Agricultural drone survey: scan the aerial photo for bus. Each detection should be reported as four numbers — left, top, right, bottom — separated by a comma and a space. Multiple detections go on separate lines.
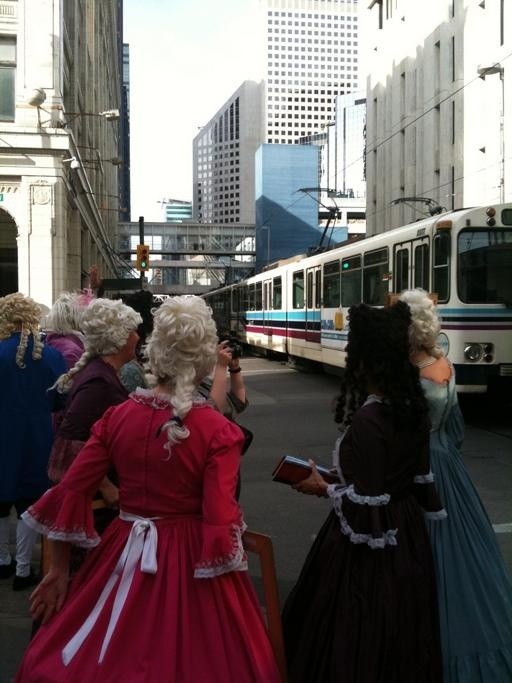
198, 200, 511, 396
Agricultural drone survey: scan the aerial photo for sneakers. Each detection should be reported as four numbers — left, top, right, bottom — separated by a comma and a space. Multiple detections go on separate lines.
0, 557, 16, 579
13, 571, 39, 591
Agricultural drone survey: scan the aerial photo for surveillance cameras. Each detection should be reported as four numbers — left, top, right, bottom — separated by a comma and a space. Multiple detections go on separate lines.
70, 160, 80, 171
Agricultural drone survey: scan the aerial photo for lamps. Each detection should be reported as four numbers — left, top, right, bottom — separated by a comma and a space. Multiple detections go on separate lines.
80, 153, 124, 166
25, 86, 47, 126
63, 107, 121, 121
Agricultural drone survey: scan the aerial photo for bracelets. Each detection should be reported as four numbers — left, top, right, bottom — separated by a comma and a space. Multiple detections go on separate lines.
228, 366, 242, 374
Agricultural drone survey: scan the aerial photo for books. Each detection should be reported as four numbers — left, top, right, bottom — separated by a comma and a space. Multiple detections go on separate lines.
271, 454, 343, 491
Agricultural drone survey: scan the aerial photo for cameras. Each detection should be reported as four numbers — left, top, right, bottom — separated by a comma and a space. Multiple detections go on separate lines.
226, 343, 243, 357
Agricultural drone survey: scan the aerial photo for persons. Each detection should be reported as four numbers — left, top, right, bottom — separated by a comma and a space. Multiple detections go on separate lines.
17, 290, 291, 683
41, 291, 88, 384
386, 283, 511, 683
42, 297, 144, 574
116, 317, 148, 397
197, 334, 252, 503
280, 298, 449, 681
2, 289, 49, 593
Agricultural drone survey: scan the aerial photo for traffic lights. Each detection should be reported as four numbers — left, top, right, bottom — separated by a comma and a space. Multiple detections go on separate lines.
137, 244, 148, 269
